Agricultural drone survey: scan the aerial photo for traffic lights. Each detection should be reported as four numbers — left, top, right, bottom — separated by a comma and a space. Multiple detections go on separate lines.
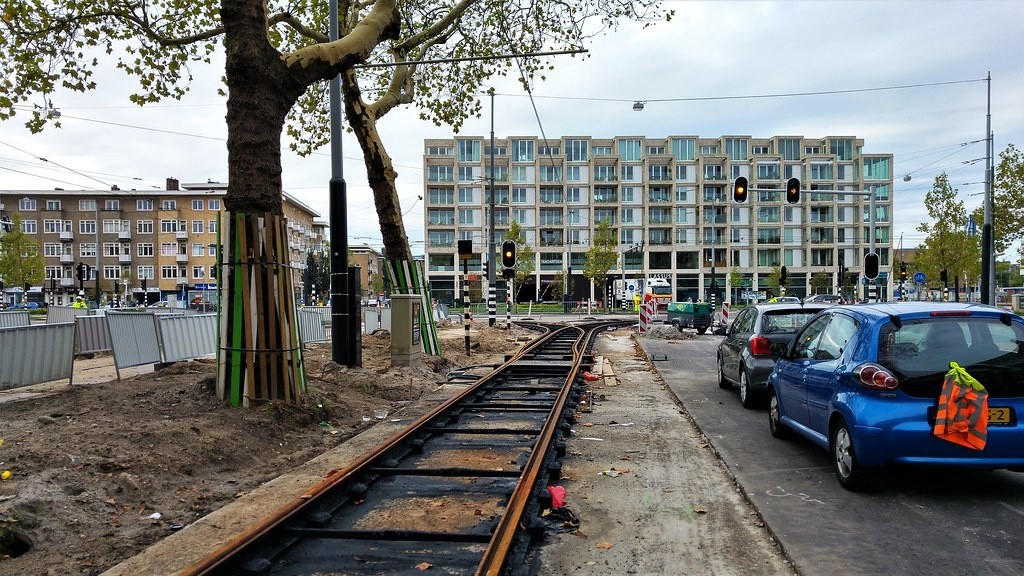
900, 266, 906, 280
458, 240, 472, 254
141, 280, 146, 290
502, 240, 516, 268
941, 270, 947, 281
734, 176, 748, 204
625, 281, 628, 290
864, 252, 881, 281
115, 283, 119, 292
787, 177, 800, 205
82, 264, 91, 281
483, 260, 488, 280
1, 215, 11, 232
640, 239, 645, 253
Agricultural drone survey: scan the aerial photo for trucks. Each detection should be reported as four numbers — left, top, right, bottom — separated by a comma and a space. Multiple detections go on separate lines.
612, 276, 673, 312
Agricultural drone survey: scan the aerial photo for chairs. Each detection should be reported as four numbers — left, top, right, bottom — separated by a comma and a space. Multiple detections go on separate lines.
925, 322, 967, 348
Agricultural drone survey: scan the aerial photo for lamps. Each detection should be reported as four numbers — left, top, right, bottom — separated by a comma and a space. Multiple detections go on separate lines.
632, 101, 644, 111
904, 174, 912, 182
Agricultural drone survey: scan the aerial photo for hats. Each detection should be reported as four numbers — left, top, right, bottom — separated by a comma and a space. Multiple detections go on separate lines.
76, 296, 82, 299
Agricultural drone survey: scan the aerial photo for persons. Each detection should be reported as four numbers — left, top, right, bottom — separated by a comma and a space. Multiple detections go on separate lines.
73, 296, 87, 308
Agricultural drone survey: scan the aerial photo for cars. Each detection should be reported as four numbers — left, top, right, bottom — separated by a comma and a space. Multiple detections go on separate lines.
64, 297, 222, 313
766, 301, 1024, 493
712, 301, 838, 409
361, 292, 391, 308
802, 293, 848, 305
761, 297, 801, 305
2, 302, 48, 310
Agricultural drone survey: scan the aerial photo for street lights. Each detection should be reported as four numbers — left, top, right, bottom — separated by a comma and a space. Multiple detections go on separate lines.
488, 86, 495, 325
